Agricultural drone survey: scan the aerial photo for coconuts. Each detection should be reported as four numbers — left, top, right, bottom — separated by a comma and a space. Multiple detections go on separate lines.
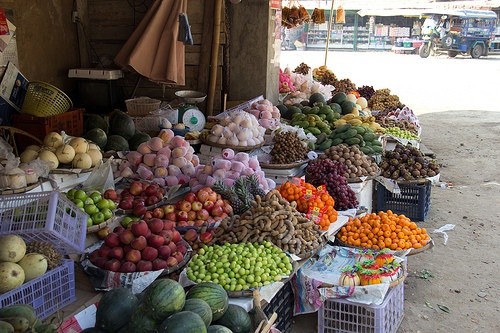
276, 92, 354, 120
81, 110, 164, 152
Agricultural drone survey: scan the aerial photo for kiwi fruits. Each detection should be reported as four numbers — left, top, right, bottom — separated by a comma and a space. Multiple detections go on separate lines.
314, 144, 376, 179
374, 115, 419, 134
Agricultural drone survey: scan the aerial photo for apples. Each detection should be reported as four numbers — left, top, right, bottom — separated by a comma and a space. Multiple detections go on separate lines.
206, 99, 281, 146
68, 180, 233, 272
113, 129, 275, 194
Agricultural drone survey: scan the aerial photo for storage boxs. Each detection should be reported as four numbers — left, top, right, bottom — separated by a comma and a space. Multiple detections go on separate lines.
263, 279, 295, 333
199, 143, 225, 158
186, 139, 202, 154
0, 258, 76, 321
412, 29, 421, 36
394, 40, 404, 47
413, 41, 423, 47
317, 278, 406, 333
74, 78, 120, 114
318, 21, 329, 31
10, 108, 87, 148
371, 180, 432, 223
260, 161, 308, 185
334, 23, 343, 30
67, 68, 122, 81
0, 190, 90, 257
296, 43, 307, 51
403, 41, 413, 47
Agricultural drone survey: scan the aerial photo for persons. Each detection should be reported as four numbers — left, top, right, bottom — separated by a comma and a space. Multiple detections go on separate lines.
437, 19, 450, 39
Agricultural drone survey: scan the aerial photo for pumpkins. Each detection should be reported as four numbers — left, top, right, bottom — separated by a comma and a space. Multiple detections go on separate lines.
339, 248, 402, 286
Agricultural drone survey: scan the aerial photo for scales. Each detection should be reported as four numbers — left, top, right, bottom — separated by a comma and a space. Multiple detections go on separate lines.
174, 90, 207, 132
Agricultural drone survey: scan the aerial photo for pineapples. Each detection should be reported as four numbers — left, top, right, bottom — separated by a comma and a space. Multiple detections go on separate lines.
213, 175, 265, 213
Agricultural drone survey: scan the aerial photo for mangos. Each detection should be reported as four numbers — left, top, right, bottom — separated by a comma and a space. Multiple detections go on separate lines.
290, 112, 385, 154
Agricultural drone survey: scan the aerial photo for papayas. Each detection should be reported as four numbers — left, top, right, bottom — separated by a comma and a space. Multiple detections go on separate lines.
0, 305, 64, 333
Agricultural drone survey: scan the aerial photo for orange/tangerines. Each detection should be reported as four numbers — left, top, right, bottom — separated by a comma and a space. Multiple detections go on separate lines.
276, 179, 338, 230
337, 210, 429, 250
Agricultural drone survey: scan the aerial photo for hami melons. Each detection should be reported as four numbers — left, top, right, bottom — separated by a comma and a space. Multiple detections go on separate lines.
0, 235, 48, 294
5, 132, 103, 188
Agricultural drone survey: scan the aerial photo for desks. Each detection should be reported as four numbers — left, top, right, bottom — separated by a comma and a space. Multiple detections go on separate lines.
228, 214, 350, 312
347, 142, 397, 214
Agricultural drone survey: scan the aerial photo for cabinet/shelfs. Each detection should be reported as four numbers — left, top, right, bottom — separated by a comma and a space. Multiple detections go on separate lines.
342, 29, 354, 49
306, 29, 342, 49
357, 28, 369, 50
369, 35, 412, 52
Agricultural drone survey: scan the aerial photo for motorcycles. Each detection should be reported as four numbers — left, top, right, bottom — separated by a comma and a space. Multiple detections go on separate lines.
418, 9, 498, 59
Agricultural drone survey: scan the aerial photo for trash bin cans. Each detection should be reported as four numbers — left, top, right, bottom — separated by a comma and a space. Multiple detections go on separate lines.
22, 81, 73, 117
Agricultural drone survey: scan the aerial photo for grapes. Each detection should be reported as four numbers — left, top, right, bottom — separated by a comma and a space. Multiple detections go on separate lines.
304, 160, 359, 211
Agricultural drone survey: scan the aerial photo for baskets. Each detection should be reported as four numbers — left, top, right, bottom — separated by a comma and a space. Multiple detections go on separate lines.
0, 191, 89, 254
0, 259, 77, 321
20, 81, 74, 119
250, 279, 294, 333
125, 98, 161, 116
378, 181, 431, 222
317, 281, 406, 333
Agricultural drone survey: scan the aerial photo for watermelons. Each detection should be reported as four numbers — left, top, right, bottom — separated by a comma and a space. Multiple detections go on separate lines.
80, 279, 252, 333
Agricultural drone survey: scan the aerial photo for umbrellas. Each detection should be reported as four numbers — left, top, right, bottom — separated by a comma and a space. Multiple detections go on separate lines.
113, 0, 187, 99
